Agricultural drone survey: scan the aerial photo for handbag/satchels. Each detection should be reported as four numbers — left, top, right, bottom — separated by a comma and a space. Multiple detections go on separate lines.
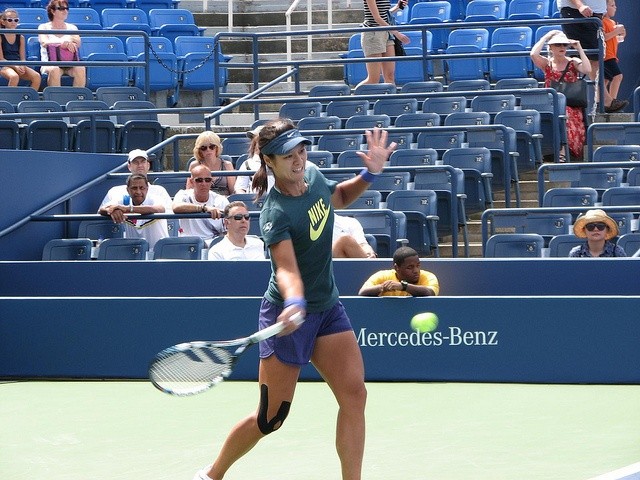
387, 10, 407, 56
550, 60, 587, 108
47, 44, 80, 61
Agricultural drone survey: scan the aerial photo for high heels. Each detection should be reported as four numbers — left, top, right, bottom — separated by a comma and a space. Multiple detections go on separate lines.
559, 154, 566, 162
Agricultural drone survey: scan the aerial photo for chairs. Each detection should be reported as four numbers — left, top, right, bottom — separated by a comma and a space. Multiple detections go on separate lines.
373, 98, 416, 115
318, 135, 364, 151
396, 113, 440, 128
133, 0, 177, 17
410, 1, 452, 24
325, 174, 356, 183
97, 238, 148, 260
467, 127, 522, 209
617, 234, 640, 257
9, 8, 46, 38
306, 151, 332, 167
96, 86, 145, 107
534, 26, 569, 80
252, 119, 274, 128
227, 195, 266, 236
400, 82, 442, 93
43, 87, 92, 106
493, 79, 538, 90
308, 85, 349, 97
64, 8, 102, 37
326, 100, 368, 118
362, 234, 377, 257
73, 119, 119, 155
348, 191, 380, 210
297, 117, 341, 130
0, 1, 30, 12
405, 141, 423, 143
391, 3, 409, 25
0, 86, 40, 104
447, 80, 491, 91
444, 111, 491, 126
345, 115, 390, 128
442, 148, 494, 209
388, 131, 414, 151
394, 30, 432, 85
27, 120, 68, 152
490, 27, 533, 78
166, 219, 179, 237
602, 187, 640, 205
549, 234, 588, 258
515, 213, 573, 237
388, 149, 437, 169
386, 190, 438, 259
445, 28, 488, 79
423, 97, 466, 114
101, 9, 151, 55
576, 212, 634, 235
417, 132, 463, 150
123, 121, 162, 172
465, 0, 507, 21
65, 100, 109, 125
152, 235, 205, 260
0, 120, 19, 150
220, 139, 251, 156
187, 155, 232, 170
552, 1, 560, 17
26, 37, 75, 92
208, 234, 260, 248
303, 135, 315, 145
592, 144, 640, 163
484, 233, 543, 259
508, 0, 550, 20
235, 154, 251, 170
114, 101, 158, 126
493, 109, 543, 166
340, 32, 368, 84
354, 211, 406, 250
471, 95, 513, 112
78, 219, 123, 241
40, 1, 79, 9
177, 36, 228, 90
0, 100, 15, 122
77, 37, 129, 87
18, 100, 62, 124
355, 82, 397, 96
368, 172, 411, 190
124, 36, 177, 91
339, 151, 366, 169
569, 168, 623, 186
626, 166, 640, 186
321, 151, 323, 154
279, 104, 321, 120
42, 237, 92, 261
152, 176, 189, 202
520, 92, 570, 166
542, 186, 598, 207
149, 8, 199, 50
90, 0, 127, 14
414, 169, 470, 258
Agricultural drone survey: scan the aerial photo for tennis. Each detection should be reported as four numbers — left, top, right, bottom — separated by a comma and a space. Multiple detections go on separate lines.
410, 311, 439, 335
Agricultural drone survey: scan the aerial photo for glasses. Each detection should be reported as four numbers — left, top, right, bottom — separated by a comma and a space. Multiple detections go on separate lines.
227, 213, 249, 220
3, 18, 19, 22
585, 223, 608, 231
551, 44, 567, 47
199, 145, 216, 151
194, 177, 212, 182
55, 6, 69, 11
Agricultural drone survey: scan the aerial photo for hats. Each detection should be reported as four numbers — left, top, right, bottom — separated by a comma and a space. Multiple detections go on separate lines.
128, 149, 148, 164
573, 210, 619, 240
260, 127, 312, 155
548, 31, 571, 44
247, 125, 265, 138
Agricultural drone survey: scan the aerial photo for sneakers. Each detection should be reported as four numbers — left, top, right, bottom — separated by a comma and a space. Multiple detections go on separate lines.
604, 98, 628, 112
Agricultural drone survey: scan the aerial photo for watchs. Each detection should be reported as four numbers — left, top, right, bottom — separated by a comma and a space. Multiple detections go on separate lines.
402, 279, 408, 290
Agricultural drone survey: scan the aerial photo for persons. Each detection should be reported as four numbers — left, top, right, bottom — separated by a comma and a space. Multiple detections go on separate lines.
208, 202, 264, 260
190, 131, 236, 194
328, 212, 373, 254
99, 150, 172, 213
359, 248, 439, 298
571, 209, 627, 257
531, 31, 593, 157
0, 12, 41, 91
599, 0, 624, 105
39, 1, 85, 88
238, 126, 275, 194
354, 0, 409, 87
105, 176, 173, 245
557, 2, 625, 111
193, 121, 396, 480
169, 166, 233, 239
